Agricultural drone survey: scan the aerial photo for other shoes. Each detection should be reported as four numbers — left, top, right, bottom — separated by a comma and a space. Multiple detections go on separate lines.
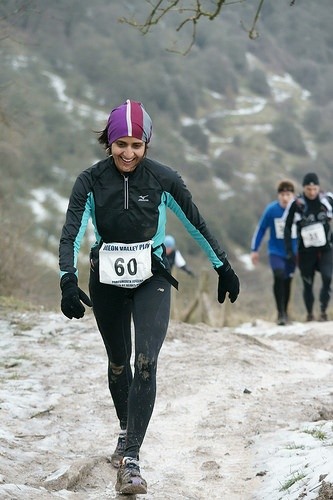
277, 313, 285, 325
321, 307, 328, 321
307, 313, 312, 321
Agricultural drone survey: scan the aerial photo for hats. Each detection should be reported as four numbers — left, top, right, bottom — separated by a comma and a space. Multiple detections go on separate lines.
303, 173, 320, 186
164, 234, 176, 248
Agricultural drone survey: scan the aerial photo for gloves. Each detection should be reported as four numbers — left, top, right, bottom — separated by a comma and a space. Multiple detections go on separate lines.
287, 250, 296, 260
60, 274, 95, 319
214, 261, 240, 304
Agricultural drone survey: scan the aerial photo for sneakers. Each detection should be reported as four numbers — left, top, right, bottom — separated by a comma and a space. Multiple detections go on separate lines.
111, 437, 126, 468
115, 463, 147, 494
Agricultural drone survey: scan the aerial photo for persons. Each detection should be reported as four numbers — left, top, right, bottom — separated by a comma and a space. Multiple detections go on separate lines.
58, 99, 240, 494
281, 172, 333, 322
165, 235, 192, 275
250, 180, 299, 324
